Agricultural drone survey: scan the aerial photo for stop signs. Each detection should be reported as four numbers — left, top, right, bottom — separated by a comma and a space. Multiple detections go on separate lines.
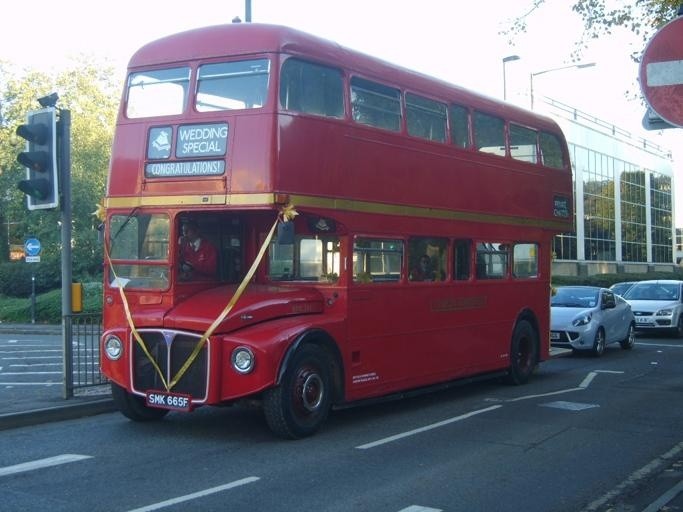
640, 15, 682, 126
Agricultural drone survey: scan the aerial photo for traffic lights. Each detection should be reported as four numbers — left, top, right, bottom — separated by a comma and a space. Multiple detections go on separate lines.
16, 106, 61, 212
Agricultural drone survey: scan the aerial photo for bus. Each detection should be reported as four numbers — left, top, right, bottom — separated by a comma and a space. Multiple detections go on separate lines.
98, 17, 574, 440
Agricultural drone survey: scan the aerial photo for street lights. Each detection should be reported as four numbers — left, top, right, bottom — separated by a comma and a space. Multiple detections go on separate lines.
503, 55, 595, 113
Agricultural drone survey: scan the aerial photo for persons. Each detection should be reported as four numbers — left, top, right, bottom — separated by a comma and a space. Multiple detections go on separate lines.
409, 254, 444, 280
176, 221, 217, 280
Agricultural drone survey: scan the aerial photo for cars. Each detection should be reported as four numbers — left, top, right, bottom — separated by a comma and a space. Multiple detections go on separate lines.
551, 279, 683, 357
